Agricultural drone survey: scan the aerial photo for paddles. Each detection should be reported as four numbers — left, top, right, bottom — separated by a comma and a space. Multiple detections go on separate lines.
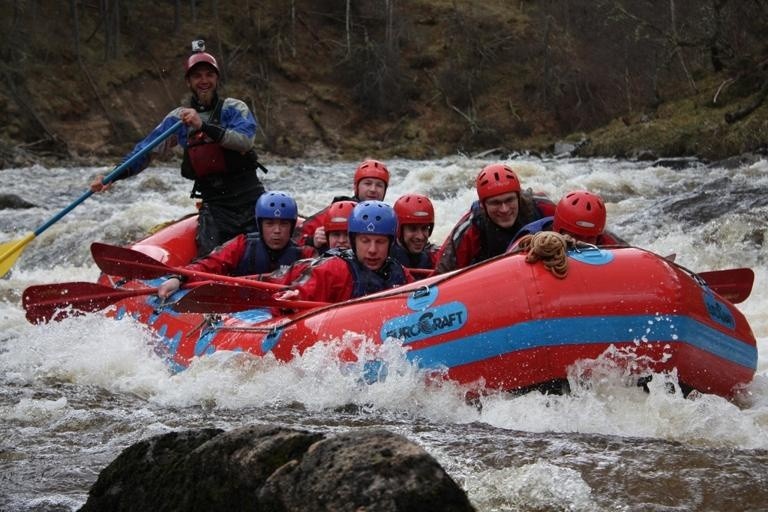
695, 265, 754, 304
22, 272, 211, 328
0, 117, 189, 269
88, 242, 285, 291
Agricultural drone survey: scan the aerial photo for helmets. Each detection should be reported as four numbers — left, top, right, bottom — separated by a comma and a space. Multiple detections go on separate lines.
347, 200, 399, 256
476, 165, 521, 202
185, 52, 220, 74
552, 192, 606, 237
354, 160, 389, 196
255, 191, 297, 239
393, 195, 434, 247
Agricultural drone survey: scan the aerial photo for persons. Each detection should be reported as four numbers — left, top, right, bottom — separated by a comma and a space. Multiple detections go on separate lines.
428, 162, 557, 277
270, 199, 417, 318
388, 194, 443, 280
157, 189, 320, 301
290, 159, 391, 257
89, 50, 266, 259
505, 190, 630, 253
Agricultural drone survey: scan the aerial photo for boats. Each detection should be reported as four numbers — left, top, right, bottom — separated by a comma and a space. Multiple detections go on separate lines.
97, 212, 758, 414
